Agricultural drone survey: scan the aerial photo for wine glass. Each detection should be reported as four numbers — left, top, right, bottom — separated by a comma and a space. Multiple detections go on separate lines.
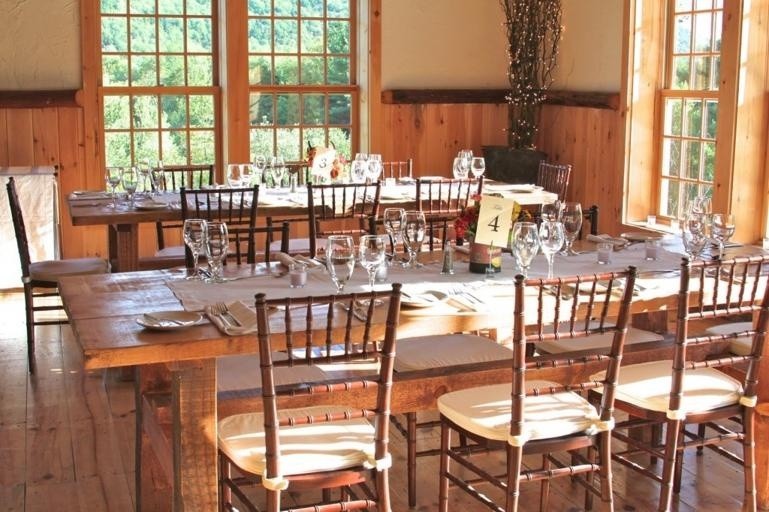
510, 197, 583, 290
227, 156, 285, 203
452, 149, 485, 186
104, 158, 164, 209
682, 213, 736, 275
358, 235, 385, 307
382, 208, 426, 270
351, 153, 383, 193
326, 235, 356, 295
183, 219, 229, 283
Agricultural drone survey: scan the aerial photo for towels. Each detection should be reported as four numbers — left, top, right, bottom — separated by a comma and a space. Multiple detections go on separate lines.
205, 301, 258, 335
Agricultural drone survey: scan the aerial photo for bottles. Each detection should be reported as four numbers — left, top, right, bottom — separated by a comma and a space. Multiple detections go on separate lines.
288, 175, 295, 192
441, 240, 454, 275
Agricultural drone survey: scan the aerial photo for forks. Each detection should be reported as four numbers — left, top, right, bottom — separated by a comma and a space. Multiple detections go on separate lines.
210, 300, 242, 327
448, 287, 485, 305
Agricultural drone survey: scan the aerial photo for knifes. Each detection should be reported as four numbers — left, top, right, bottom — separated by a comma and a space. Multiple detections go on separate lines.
337, 301, 367, 323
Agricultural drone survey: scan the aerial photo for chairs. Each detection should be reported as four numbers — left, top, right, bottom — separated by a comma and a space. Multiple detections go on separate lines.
184, 220, 292, 264
6, 176, 110, 373
588, 256, 769, 512
515, 205, 659, 480
368, 216, 514, 504
436, 266, 635, 512
218, 282, 402, 512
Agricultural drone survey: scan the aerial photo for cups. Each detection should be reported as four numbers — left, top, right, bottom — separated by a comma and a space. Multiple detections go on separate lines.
596, 242, 613, 264
647, 215, 656, 225
645, 240, 660, 261
289, 263, 308, 288
671, 220, 679, 230
386, 178, 395, 190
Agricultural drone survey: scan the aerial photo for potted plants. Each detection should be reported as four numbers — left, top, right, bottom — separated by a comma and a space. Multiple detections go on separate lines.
482, 0, 565, 185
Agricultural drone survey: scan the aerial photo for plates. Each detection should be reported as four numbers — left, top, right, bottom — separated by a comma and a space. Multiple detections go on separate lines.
400, 290, 450, 308
135, 311, 203, 331
620, 232, 663, 241
